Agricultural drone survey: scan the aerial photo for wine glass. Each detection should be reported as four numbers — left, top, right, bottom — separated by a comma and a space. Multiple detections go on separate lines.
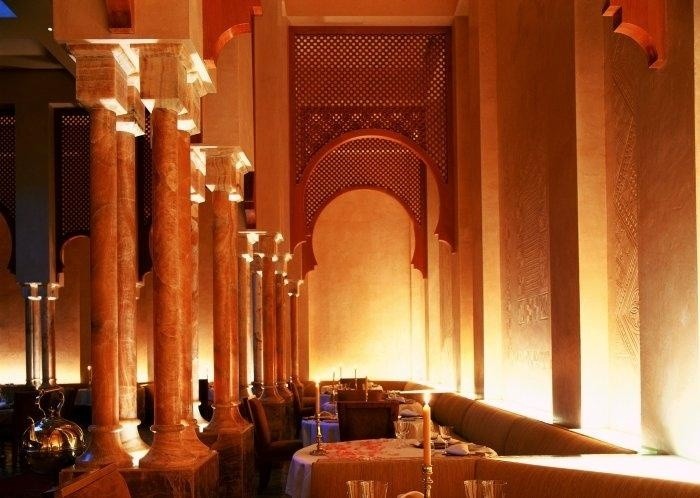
439, 425, 454, 456
393, 420, 411, 449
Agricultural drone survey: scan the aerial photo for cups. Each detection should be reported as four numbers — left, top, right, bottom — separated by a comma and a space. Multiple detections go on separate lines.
345, 480, 389, 498
463, 478, 509, 498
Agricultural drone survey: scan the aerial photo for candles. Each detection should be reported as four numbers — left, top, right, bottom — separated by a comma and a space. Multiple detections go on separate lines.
419, 390, 432, 467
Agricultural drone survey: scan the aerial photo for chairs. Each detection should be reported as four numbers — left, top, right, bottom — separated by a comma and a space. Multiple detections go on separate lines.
241, 375, 400, 498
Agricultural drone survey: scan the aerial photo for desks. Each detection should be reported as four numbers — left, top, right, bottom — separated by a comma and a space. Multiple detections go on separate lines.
290, 439, 498, 498
